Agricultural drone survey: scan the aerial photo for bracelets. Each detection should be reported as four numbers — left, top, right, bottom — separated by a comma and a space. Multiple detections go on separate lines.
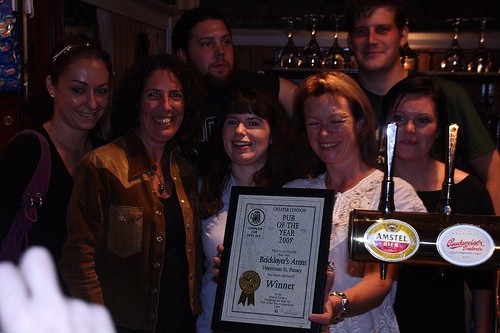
330, 291, 350, 325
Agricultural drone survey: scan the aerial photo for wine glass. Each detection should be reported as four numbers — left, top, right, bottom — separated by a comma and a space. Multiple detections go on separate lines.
445, 18, 468, 72
323, 14, 348, 69
301, 15, 325, 68
400, 18, 417, 70
279, 17, 302, 68
471, 17, 494, 73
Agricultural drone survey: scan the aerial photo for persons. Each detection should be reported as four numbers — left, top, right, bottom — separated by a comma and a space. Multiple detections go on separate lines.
345, 0, 500, 216
376, 77, 496, 333
195, 87, 297, 333
171, 8, 299, 145
212, 71, 429, 333
60, 54, 202, 332
0, 34, 113, 299
